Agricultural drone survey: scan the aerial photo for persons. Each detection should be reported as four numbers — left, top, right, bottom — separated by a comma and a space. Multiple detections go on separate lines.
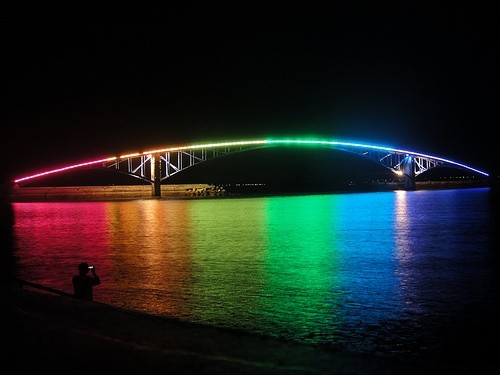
72, 262, 102, 301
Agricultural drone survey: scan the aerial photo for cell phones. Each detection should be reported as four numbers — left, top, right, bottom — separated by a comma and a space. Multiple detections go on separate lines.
88, 266, 94, 270
